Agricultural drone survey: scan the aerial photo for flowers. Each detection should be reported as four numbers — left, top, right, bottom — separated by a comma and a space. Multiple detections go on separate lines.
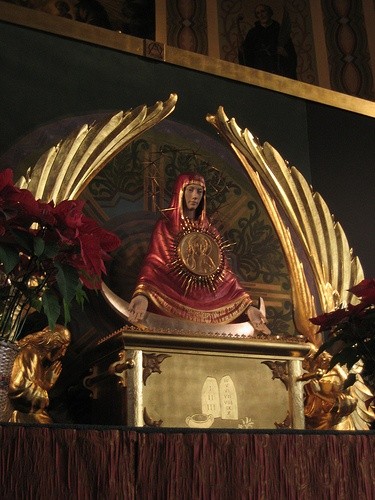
308, 277, 375, 431
0, 168, 124, 344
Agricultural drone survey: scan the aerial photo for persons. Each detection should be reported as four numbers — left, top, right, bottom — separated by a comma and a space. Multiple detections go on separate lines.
126, 173, 272, 336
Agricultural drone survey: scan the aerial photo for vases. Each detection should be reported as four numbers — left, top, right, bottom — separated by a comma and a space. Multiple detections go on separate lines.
0, 323, 71, 425
